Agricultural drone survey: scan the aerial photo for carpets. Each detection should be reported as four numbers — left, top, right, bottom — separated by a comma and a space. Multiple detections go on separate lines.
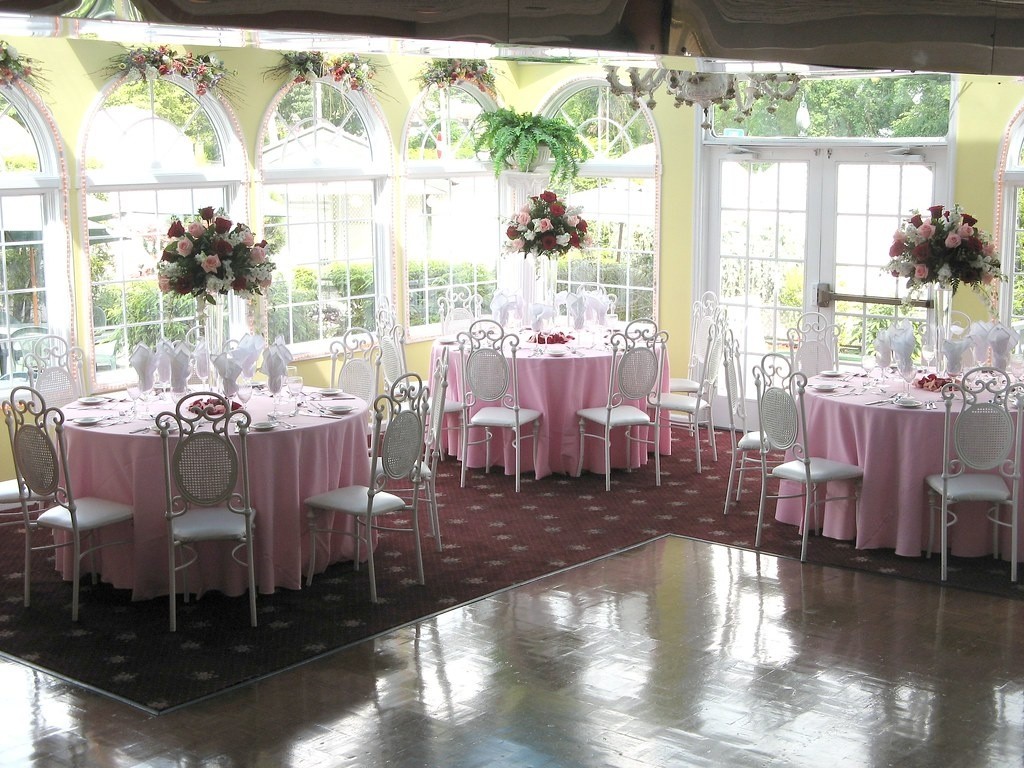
0, 427, 1021, 718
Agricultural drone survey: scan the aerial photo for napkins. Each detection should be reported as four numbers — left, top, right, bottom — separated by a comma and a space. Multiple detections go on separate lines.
129, 287, 1022, 395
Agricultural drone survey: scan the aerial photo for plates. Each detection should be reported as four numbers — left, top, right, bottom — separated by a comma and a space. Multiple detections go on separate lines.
327, 404, 352, 415
895, 398, 922, 409
148, 423, 177, 433
249, 422, 278, 431
72, 416, 101, 426
548, 351, 566, 356
78, 396, 104, 405
319, 388, 344, 396
812, 384, 838, 392
440, 337, 457, 344
821, 370, 842, 376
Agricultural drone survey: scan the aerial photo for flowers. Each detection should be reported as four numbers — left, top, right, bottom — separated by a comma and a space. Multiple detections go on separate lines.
86, 42, 247, 108
157, 205, 277, 305
885, 201, 1010, 305
260, 50, 399, 103
410, 57, 506, 104
496, 191, 593, 257
0, 40, 53, 94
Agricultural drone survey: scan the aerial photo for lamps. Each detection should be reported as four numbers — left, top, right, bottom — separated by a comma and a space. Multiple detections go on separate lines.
604, 65, 805, 129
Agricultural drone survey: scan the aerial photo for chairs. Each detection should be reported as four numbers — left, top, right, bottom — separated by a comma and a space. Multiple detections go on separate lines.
25, 336, 86, 434
329, 283, 1024, 633
2, 386, 134, 622
305, 374, 428, 588
155, 391, 259, 631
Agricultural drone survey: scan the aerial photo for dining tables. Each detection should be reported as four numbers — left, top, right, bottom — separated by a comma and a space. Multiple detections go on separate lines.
775, 366, 1024, 566
426, 319, 672, 480
50, 380, 370, 604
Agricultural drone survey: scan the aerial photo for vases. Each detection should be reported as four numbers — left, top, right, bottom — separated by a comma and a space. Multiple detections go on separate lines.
526, 247, 558, 324
200, 287, 227, 392
925, 282, 954, 374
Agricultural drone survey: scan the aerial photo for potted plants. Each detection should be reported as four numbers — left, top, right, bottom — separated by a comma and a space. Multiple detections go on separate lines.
470, 106, 590, 187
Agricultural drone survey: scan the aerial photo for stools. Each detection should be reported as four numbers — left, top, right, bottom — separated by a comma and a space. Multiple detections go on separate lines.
0, 477, 55, 533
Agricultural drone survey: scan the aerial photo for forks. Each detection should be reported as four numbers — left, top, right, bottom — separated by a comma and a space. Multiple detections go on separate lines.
812, 369, 938, 410
65, 391, 355, 434
438, 324, 586, 357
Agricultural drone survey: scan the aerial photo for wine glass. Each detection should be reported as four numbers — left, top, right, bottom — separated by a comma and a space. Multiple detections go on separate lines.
125, 367, 304, 422
490, 308, 618, 351
862, 346, 1023, 403
